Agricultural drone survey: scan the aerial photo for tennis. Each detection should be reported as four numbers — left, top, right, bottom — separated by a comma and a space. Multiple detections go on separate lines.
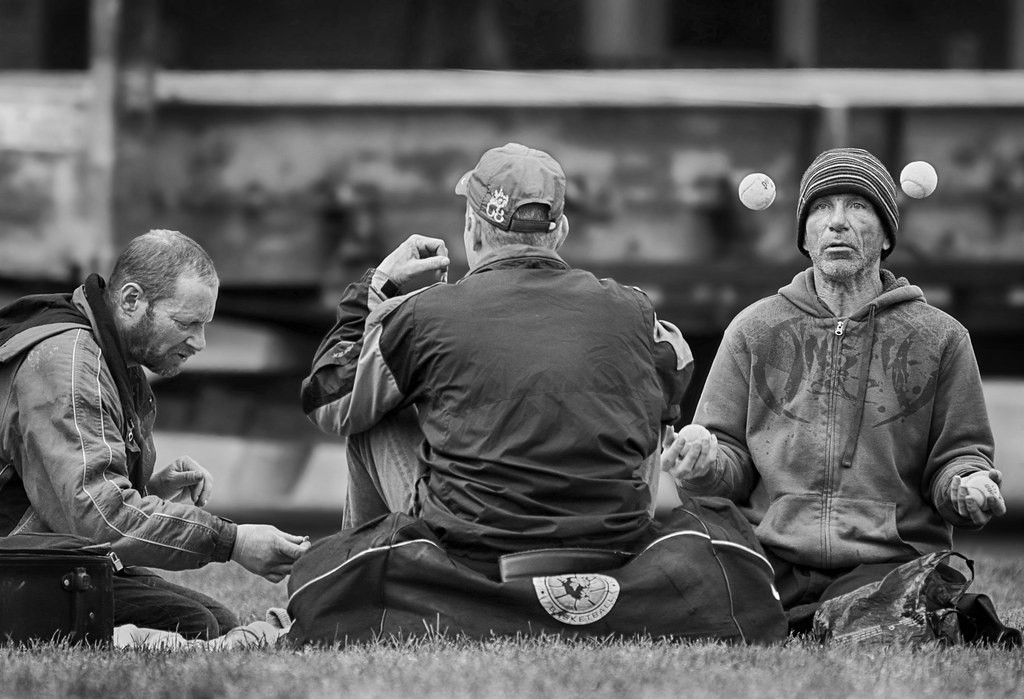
738, 171, 776, 211
963, 476, 1000, 510
899, 160, 939, 199
677, 423, 712, 459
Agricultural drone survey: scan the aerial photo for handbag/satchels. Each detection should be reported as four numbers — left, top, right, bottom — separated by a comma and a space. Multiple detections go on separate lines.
0, 533, 122, 649
288, 496, 789, 648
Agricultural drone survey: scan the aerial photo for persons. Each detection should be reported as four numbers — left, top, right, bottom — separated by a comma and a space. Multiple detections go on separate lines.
0, 228, 313, 650
301, 143, 697, 579
659, 147, 1007, 637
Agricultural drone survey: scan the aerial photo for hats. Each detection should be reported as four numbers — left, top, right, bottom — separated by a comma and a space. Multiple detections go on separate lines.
795, 146, 900, 261
455, 143, 567, 233
